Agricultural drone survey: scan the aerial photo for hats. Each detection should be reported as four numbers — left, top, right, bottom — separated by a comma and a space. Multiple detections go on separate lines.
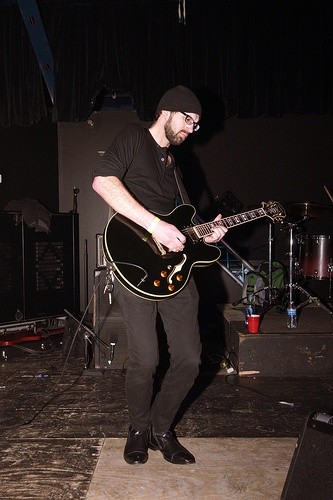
157, 86, 202, 116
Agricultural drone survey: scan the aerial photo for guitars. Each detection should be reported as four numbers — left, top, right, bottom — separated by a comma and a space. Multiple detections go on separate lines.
103, 200, 286, 301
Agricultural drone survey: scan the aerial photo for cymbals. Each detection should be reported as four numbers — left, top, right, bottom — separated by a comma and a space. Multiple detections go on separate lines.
287, 203, 333, 218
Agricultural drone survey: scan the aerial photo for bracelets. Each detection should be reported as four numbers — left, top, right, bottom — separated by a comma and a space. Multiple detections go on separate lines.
147, 217, 161, 233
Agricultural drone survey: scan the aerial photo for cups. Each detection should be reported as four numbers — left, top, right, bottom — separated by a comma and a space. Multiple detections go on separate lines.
247, 314, 260, 333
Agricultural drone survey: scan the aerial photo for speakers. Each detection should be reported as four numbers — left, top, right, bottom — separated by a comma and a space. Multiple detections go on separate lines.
94, 269, 162, 371
280, 411, 333, 500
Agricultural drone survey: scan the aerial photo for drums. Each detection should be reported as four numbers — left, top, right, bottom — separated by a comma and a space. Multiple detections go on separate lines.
292, 234, 333, 280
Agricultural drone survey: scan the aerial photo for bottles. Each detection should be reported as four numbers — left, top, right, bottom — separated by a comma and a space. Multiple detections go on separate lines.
244, 295, 256, 325
287, 301, 297, 328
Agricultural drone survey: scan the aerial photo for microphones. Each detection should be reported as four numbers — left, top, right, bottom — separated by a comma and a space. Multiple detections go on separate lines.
110, 335, 117, 359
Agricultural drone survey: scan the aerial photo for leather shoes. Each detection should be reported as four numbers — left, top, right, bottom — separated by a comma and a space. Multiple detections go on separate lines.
148, 432, 196, 464
124, 424, 148, 465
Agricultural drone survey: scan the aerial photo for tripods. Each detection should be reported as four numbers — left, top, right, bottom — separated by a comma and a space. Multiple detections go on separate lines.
235, 223, 333, 316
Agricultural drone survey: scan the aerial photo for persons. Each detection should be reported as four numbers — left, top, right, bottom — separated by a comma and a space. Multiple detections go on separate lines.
92, 86, 227, 464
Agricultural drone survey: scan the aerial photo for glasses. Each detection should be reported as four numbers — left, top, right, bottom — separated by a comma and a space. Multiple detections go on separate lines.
180, 111, 200, 132
